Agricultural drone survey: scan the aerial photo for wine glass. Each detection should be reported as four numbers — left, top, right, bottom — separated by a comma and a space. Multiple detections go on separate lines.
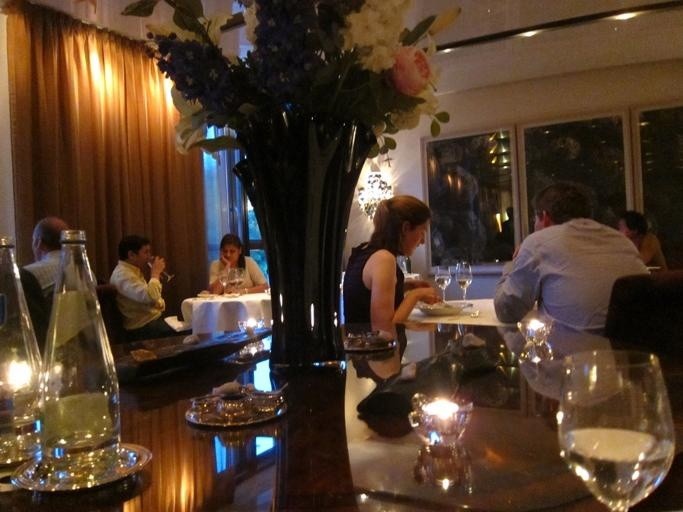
219, 268, 245, 298
434, 265, 452, 301
455, 263, 473, 301
147, 256, 175, 283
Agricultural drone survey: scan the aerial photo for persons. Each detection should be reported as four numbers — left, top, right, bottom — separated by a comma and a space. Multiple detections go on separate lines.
0, 217, 96, 355
615, 211, 668, 275
343, 323, 440, 398
484, 178, 652, 337
497, 325, 628, 432
341, 192, 443, 326
107, 233, 193, 338
207, 232, 269, 296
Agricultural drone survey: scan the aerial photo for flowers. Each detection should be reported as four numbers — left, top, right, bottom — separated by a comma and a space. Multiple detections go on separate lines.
119, 1, 465, 168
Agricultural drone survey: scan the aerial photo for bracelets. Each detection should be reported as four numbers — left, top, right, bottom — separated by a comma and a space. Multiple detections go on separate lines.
243, 287, 248, 293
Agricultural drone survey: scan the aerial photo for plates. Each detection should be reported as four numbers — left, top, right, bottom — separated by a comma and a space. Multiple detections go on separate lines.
185, 396, 288, 427
197, 294, 217, 299
343, 336, 396, 350
10, 442, 153, 492
416, 302, 473, 316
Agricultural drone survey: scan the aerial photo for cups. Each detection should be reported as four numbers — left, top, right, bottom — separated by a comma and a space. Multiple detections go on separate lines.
556, 348, 676, 512
407, 391, 474, 449
517, 319, 556, 347
192, 390, 284, 422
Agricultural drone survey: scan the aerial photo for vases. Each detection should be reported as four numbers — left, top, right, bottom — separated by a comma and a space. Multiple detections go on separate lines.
231, 109, 378, 376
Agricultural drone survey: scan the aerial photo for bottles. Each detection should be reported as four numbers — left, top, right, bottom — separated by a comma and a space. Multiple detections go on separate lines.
0, 230, 121, 481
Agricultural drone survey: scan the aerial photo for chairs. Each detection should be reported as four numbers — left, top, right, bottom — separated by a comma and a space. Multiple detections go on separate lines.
94, 286, 168, 338
606, 274, 683, 361
